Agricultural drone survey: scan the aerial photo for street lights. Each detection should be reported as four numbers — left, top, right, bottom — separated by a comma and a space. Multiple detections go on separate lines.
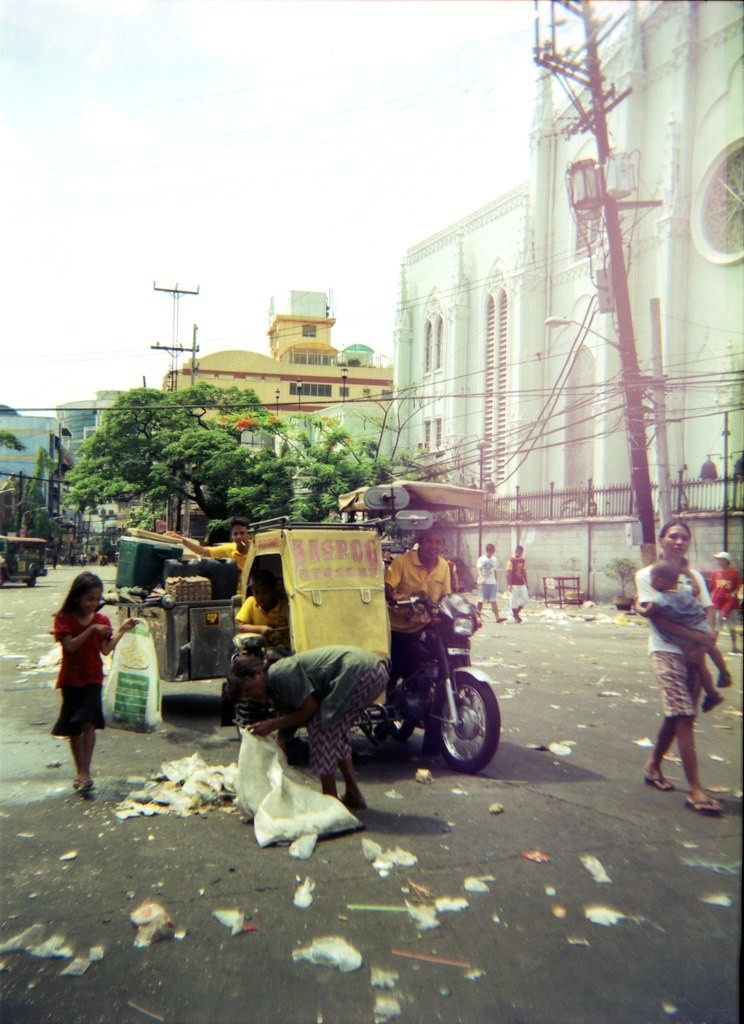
545, 316, 658, 566
342, 369, 348, 404
296, 378, 302, 411
275, 388, 280, 416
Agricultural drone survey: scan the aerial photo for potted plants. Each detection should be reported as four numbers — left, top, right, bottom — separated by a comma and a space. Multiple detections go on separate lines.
605, 559, 636, 611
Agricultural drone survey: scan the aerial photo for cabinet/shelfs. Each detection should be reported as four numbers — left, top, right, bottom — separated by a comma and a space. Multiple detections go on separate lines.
542, 576, 581, 610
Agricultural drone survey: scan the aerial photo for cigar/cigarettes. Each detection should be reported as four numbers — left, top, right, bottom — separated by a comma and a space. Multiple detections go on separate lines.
240, 540, 246, 547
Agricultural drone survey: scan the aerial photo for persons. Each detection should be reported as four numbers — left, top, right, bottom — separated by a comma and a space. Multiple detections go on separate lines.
706, 551, 742, 653
234, 567, 290, 633
51, 573, 140, 794
634, 520, 723, 815
6, 541, 40, 575
382, 527, 460, 692
505, 546, 528, 624
225, 645, 390, 810
42, 543, 119, 569
632, 561, 732, 712
476, 544, 507, 623
163, 516, 254, 596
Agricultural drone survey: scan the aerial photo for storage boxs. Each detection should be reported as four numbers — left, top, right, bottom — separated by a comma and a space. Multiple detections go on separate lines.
115, 541, 184, 588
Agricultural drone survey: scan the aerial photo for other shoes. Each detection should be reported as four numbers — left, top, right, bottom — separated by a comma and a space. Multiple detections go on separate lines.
396, 683, 420, 708
717, 671, 732, 688
497, 618, 507, 623
73, 779, 94, 798
729, 648, 742, 656
514, 614, 521, 622
702, 691, 724, 713
338, 793, 367, 809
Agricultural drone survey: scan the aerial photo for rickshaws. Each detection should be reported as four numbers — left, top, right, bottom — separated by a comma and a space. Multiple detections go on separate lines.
229, 480, 502, 773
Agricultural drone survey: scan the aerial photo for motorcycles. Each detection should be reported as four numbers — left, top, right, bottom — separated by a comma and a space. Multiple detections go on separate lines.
0, 536, 48, 587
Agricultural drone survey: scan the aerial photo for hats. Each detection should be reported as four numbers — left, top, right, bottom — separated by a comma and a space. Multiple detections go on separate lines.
714, 552, 731, 560
418, 523, 446, 545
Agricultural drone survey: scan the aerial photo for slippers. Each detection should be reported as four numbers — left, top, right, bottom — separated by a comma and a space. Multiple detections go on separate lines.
643, 773, 674, 791
685, 797, 723, 816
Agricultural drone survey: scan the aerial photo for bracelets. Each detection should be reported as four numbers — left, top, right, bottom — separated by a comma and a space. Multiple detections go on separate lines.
269, 719, 275, 731
